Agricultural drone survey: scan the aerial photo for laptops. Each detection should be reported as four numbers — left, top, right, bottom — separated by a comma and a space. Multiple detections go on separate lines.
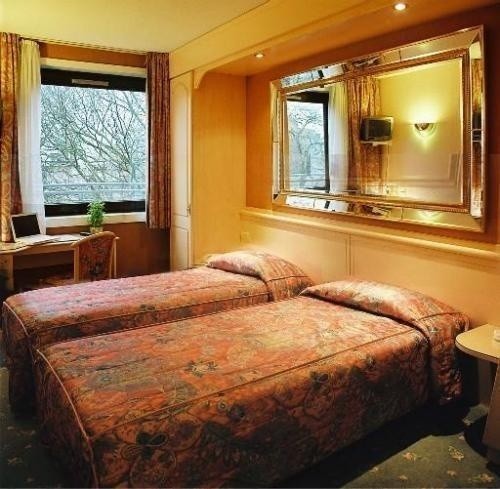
10, 213, 58, 247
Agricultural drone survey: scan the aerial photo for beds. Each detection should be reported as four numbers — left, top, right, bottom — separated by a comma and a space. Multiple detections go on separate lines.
33, 277, 471, 487
3, 252, 314, 401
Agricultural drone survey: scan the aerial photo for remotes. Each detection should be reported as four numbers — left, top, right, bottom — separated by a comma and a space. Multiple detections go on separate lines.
80, 231, 91, 236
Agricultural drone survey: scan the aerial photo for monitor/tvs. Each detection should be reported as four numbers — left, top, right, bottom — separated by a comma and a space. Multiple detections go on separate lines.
361, 116, 393, 142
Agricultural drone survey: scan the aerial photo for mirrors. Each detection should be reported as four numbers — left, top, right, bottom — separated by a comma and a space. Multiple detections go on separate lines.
268, 24, 487, 235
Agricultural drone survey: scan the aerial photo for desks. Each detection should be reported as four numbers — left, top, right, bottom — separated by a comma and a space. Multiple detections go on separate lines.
0, 234, 120, 290
454, 323, 499, 466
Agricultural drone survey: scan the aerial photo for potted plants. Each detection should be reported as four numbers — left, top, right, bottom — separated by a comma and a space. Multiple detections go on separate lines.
86, 198, 106, 233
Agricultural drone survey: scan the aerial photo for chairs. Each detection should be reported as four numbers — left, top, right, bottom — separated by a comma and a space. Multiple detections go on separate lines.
71, 231, 120, 284
